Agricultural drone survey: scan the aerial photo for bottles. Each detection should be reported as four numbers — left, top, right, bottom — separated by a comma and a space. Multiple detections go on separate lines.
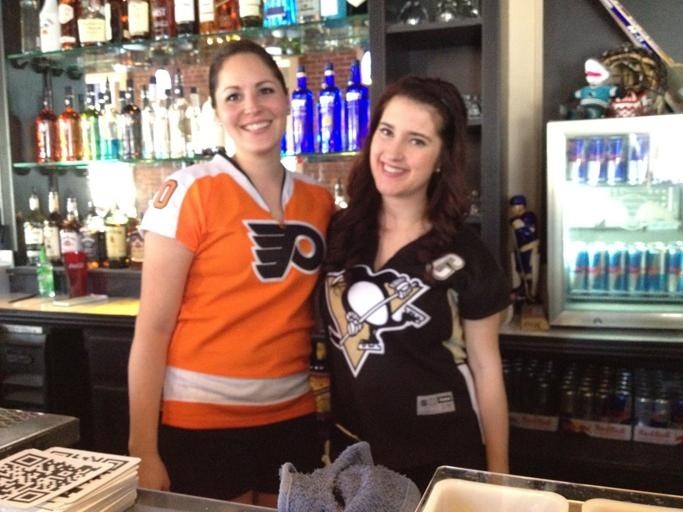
80, 84, 100, 160
128, 0, 347, 38
78, 0, 107, 47
18, 0, 41, 55
119, 76, 225, 160
60, 0, 82, 49
35, 246, 56, 298
23, 187, 146, 269
38, 0, 60, 53
96, 92, 119, 160
289, 61, 369, 152
34, 87, 56, 162
57, 86, 81, 161
105, 0, 130, 43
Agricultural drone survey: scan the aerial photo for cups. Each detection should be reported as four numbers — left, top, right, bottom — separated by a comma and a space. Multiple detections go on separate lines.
63, 252, 88, 298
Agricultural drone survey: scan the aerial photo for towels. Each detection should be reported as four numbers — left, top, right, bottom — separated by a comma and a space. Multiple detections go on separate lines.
277, 441, 422, 512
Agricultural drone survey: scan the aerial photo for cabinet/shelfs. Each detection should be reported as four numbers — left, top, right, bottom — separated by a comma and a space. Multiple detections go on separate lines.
1, 1, 370, 457
368, 0, 545, 313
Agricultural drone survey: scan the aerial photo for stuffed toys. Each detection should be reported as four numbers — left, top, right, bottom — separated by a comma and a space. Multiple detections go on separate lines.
572, 57, 623, 119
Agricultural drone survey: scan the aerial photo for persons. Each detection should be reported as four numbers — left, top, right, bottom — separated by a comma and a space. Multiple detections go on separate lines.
505, 195, 542, 301
127, 39, 344, 510
319, 75, 511, 497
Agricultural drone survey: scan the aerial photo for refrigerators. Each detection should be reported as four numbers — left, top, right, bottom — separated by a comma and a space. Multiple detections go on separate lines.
546, 113, 683, 330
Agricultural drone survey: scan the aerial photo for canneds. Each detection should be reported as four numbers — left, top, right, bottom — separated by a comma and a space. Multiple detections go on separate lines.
499, 359, 682, 426
566, 240, 683, 294
568, 134, 648, 185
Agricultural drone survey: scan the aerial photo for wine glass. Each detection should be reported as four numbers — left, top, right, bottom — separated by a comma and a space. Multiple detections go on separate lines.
433, 0, 458, 24
398, 0, 429, 26
458, 0, 480, 18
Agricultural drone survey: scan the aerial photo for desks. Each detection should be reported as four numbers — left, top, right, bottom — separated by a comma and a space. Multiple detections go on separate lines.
490, 304, 683, 496
